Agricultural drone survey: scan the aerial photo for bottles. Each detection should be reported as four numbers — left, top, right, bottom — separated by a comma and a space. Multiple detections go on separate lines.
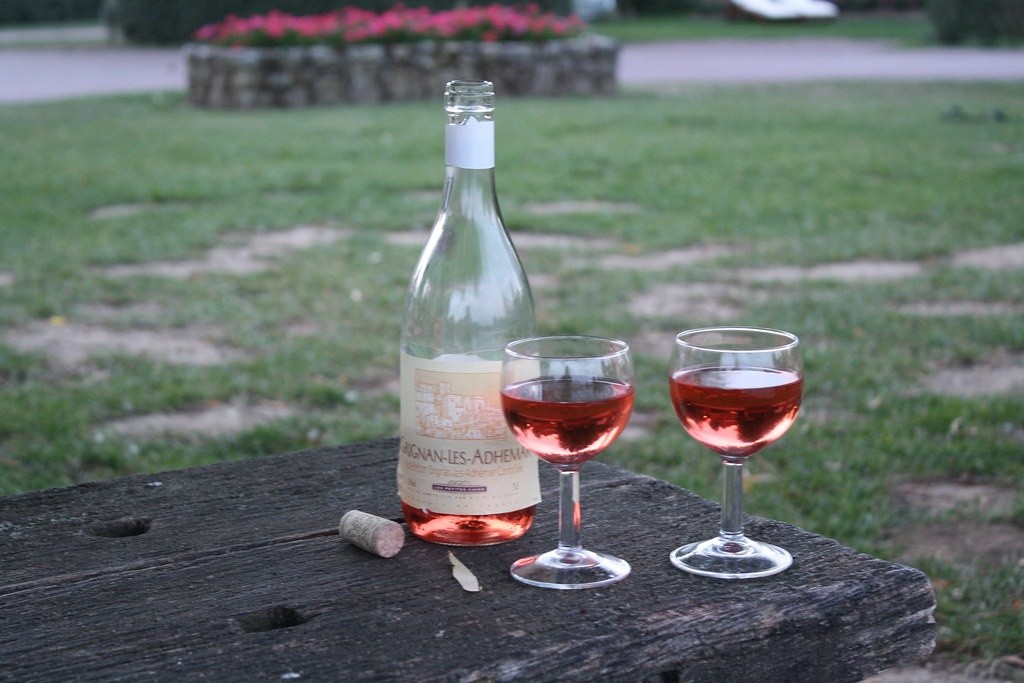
397, 80, 543, 547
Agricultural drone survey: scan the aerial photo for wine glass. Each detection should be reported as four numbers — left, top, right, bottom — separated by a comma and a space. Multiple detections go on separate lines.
669, 326, 804, 579
500, 336, 635, 590
